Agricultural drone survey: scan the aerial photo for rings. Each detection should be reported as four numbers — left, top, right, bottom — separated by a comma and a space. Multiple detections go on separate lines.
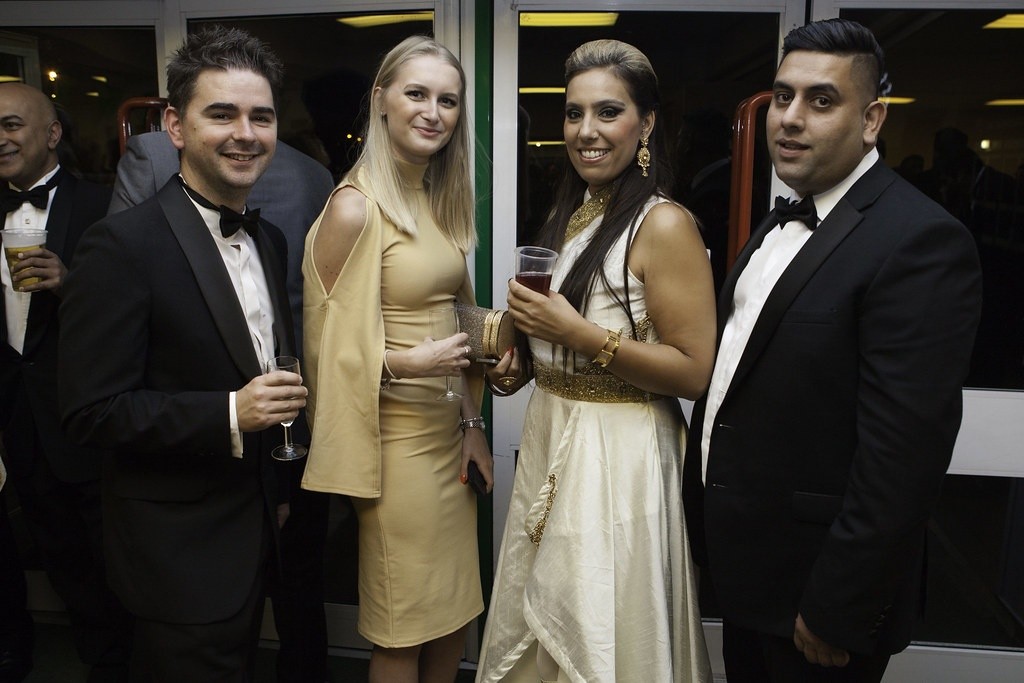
462, 346, 470, 357
498, 375, 516, 386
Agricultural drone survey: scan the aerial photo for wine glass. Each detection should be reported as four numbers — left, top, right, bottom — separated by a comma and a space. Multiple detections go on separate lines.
267, 355, 308, 460
428, 307, 463, 403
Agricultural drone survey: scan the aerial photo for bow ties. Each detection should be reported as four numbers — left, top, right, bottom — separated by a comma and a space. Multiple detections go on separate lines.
178, 176, 261, 239
0, 168, 60, 213
774, 194, 818, 231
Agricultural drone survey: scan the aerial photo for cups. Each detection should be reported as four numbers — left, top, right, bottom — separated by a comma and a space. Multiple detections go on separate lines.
514, 246, 559, 297
2, 228, 48, 292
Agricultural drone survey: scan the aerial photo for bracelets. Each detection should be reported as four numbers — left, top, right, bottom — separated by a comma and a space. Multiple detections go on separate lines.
381, 348, 401, 389
460, 416, 486, 432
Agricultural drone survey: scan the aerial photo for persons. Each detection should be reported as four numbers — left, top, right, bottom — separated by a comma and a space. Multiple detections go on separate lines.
44, 26, 334, 681
303, 35, 497, 682
684, 17, 966, 682
488, 40, 712, 683
0, 81, 105, 682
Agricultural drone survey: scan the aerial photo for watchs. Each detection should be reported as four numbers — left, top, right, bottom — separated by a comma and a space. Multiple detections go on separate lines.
591, 330, 619, 369
